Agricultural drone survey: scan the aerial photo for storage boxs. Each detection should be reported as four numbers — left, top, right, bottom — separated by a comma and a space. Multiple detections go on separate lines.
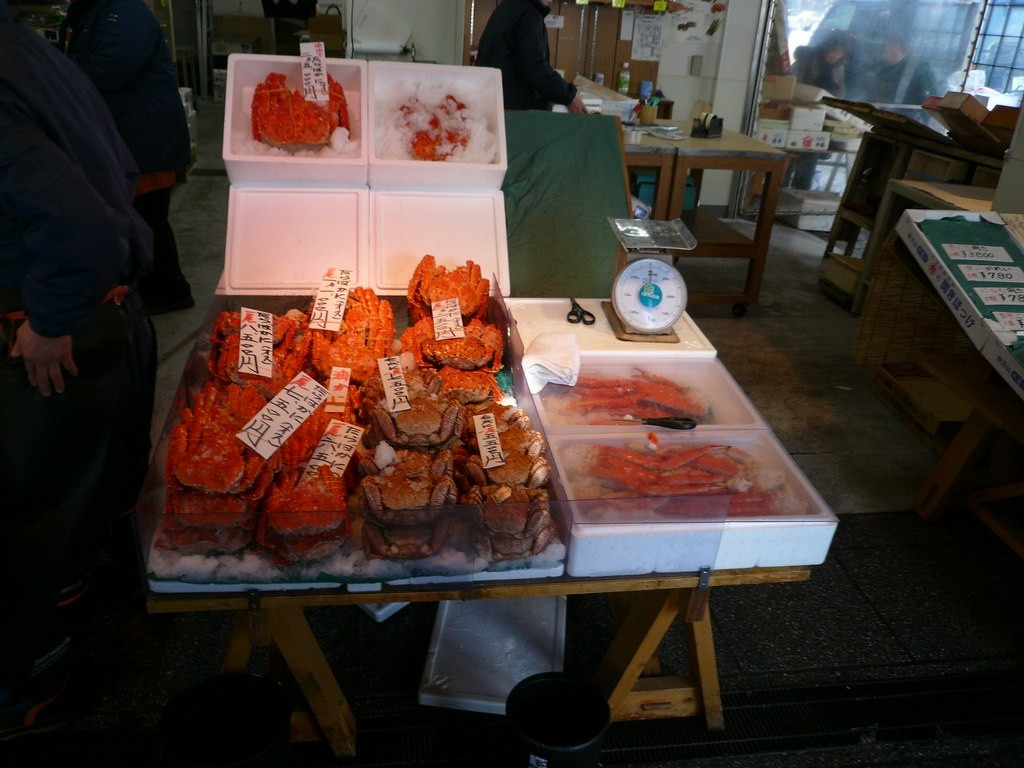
219, 52, 368, 185
504, 296, 717, 359
132, 296, 570, 591
517, 356, 772, 441
211, 36, 261, 57
213, 85, 227, 103
310, 12, 345, 51
816, 123, 1005, 315
874, 361, 969, 440
548, 428, 839, 580
214, 14, 276, 55
824, 249, 865, 297
369, 62, 508, 189
901, 151, 966, 194
212, 69, 227, 85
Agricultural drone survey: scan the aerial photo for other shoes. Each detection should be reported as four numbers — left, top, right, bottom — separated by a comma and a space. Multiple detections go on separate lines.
0, 692, 74, 743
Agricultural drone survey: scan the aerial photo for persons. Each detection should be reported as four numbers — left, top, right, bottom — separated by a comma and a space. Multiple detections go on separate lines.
474, 0, 588, 114
0, 21, 159, 740
261, 0, 318, 56
783, 31, 937, 191
56, 0, 195, 316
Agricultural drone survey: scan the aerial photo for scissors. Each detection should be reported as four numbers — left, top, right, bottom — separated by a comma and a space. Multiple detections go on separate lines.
607, 416, 697, 430
566, 290, 596, 325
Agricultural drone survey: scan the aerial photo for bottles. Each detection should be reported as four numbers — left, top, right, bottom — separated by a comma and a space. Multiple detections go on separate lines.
618, 63, 630, 94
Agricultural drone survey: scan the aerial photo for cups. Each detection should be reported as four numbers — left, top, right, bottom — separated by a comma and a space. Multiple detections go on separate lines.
639, 81, 653, 99
639, 106, 658, 126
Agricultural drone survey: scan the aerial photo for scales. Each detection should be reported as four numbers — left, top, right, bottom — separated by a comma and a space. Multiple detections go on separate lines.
600, 216, 697, 343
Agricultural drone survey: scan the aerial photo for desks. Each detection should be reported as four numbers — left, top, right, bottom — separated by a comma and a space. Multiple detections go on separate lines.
656, 118, 787, 303
141, 568, 813, 757
618, 126, 675, 219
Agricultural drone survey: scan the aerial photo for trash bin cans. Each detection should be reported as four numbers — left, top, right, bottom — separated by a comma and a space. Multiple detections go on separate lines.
505, 671, 613, 768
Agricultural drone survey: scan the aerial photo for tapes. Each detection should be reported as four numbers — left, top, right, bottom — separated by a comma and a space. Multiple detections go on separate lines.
623, 129, 642, 145
699, 112, 717, 128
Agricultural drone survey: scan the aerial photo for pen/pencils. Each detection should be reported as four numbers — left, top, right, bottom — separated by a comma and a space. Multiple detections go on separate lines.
645, 97, 654, 106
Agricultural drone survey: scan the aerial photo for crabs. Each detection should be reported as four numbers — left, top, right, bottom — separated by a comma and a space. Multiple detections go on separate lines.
400, 95, 470, 160
250, 70, 350, 149
164, 254, 808, 568
